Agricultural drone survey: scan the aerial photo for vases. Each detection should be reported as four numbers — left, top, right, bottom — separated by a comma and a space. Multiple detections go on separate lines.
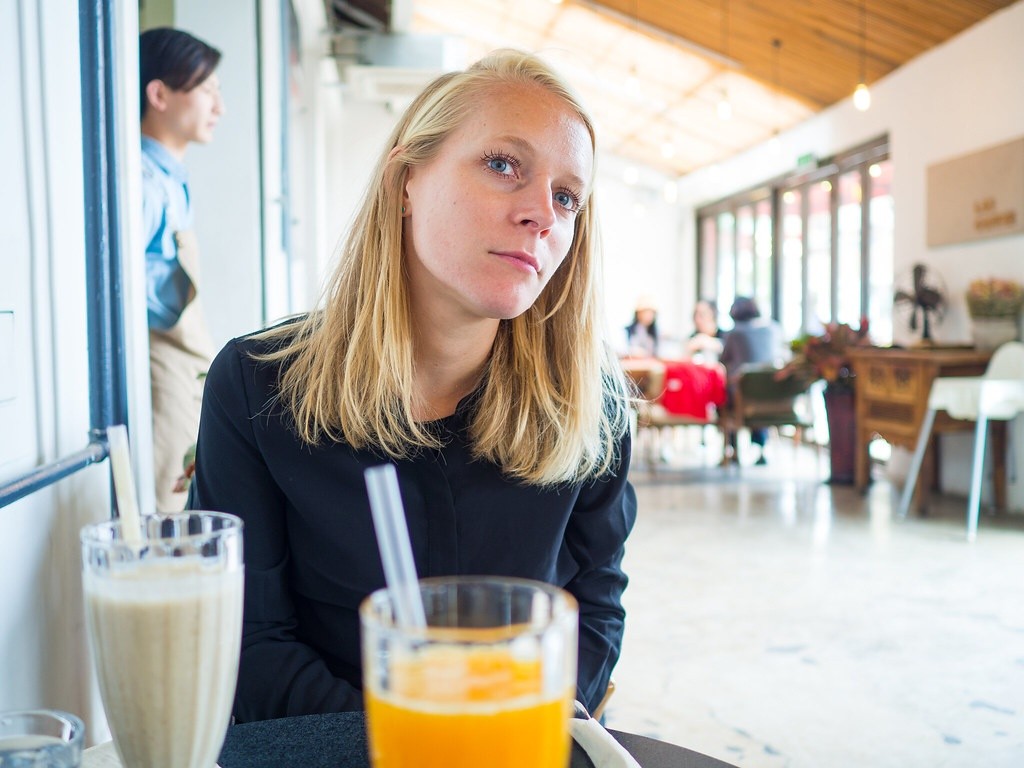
823, 392, 855, 483
970, 315, 1018, 351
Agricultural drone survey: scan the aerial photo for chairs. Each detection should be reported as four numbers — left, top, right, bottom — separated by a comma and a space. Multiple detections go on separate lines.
896, 342, 1024, 539
639, 365, 723, 470
726, 365, 802, 466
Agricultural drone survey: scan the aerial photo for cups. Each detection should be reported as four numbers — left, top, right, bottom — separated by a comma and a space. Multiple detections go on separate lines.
81, 510, 245, 768
0, 709, 84, 768
358, 576, 576, 768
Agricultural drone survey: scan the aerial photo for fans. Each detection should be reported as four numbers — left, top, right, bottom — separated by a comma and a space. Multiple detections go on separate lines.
893, 262, 949, 347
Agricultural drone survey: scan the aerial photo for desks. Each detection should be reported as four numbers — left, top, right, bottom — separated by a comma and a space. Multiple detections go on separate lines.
848, 344, 1005, 514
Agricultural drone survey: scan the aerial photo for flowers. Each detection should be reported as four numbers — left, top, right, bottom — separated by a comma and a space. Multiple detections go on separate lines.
772, 316, 872, 392
964, 277, 1024, 315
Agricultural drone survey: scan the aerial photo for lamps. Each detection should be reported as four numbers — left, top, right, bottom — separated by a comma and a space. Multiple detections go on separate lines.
853, 0, 870, 110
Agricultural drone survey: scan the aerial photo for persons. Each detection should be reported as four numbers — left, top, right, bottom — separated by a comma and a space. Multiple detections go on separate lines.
138, 27, 225, 516
625, 305, 673, 467
185, 51, 638, 726
685, 297, 785, 468
